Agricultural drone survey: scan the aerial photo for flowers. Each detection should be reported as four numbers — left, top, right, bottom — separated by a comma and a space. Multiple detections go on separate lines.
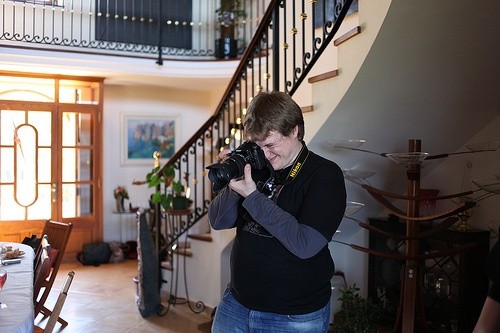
215, 0, 248, 29
115, 186, 130, 199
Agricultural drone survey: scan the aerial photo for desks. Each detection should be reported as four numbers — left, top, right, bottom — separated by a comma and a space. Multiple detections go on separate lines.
112, 211, 140, 245
0, 241, 35, 333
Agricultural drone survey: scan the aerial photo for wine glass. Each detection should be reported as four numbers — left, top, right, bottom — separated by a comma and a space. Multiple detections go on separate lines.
0, 269, 7, 309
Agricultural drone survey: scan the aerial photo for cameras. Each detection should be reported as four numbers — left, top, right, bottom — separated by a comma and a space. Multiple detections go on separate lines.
205, 141, 268, 192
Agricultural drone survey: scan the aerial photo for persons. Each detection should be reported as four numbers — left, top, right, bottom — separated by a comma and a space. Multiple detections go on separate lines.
472, 239, 500, 333
208, 90, 347, 333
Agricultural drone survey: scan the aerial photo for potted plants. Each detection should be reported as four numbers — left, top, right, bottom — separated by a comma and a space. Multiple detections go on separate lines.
146, 150, 194, 211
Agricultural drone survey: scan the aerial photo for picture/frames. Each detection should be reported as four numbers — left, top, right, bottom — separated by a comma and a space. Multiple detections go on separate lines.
118, 111, 180, 166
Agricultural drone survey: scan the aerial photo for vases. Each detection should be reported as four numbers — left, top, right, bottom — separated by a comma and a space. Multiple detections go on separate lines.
115, 196, 125, 212
216, 38, 236, 59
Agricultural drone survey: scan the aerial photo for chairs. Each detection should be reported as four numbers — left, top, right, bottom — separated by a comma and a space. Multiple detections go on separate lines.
24, 220, 75, 333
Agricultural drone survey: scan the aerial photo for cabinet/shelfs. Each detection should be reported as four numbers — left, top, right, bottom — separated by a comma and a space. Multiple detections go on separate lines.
366, 216, 490, 333
